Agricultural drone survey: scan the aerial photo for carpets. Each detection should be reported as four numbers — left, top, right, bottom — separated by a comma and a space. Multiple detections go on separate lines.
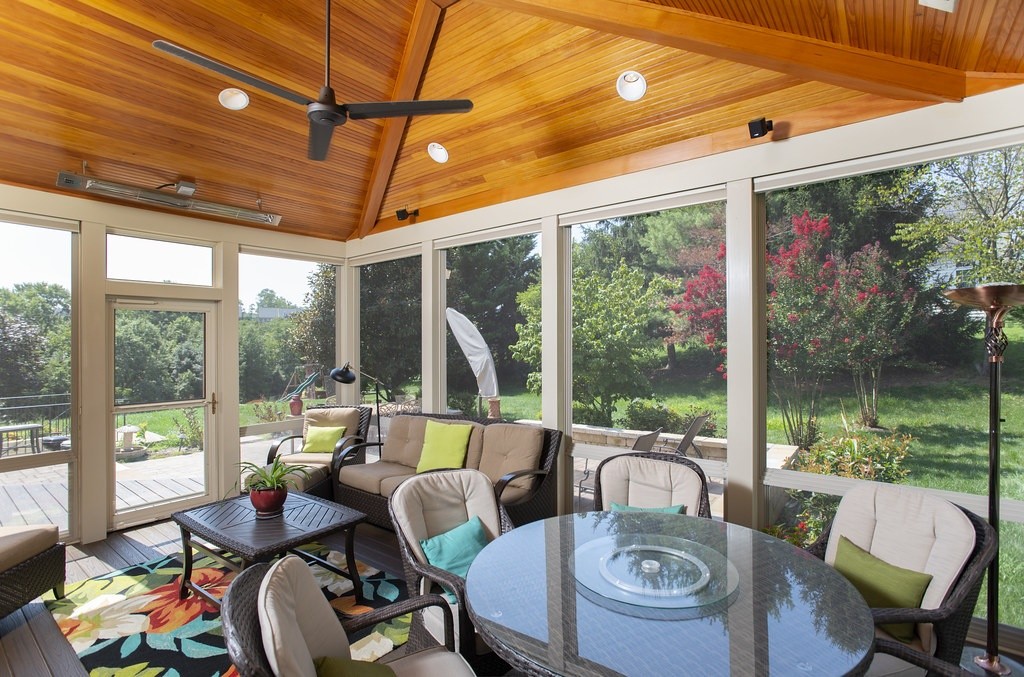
40, 542, 412, 677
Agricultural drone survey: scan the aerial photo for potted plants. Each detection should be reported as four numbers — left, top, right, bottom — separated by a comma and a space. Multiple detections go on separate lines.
218, 454, 325, 519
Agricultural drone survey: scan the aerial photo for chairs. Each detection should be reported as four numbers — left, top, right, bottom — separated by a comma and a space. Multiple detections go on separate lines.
220, 553, 477, 677
594, 453, 712, 520
650, 413, 714, 459
803, 480, 1000, 675
388, 467, 519, 666
875, 639, 974, 677
632, 427, 663, 452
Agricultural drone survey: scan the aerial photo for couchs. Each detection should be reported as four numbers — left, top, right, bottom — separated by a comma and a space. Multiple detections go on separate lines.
332, 410, 562, 527
0, 516, 67, 622
244, 405, 372, 495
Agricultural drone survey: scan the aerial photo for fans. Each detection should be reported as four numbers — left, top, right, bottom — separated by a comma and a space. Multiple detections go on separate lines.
152, 0, 474, 162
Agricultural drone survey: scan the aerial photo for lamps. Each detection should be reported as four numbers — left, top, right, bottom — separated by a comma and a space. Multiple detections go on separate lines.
396, 204, 420, 220
748, 117, 773, 138
942, 282, 1024, 677
330, 363, 385, 458
56, 171, 282, 227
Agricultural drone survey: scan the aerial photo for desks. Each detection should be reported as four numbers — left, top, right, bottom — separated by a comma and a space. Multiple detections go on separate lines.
463, 510, 875, 677
0, 423, 45, 456
171, 487, 368, 609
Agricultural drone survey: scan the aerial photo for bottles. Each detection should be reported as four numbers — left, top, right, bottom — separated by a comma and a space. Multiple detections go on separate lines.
289, 395, 303, 416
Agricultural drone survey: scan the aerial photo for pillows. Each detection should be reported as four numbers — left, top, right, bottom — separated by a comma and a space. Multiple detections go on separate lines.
302, 423, 347, 452
312, 653, 396, 677
419, 513, 488, 604
610, 501, 684, 514
416, 419, 473, 474
831, 534, 934, 639
